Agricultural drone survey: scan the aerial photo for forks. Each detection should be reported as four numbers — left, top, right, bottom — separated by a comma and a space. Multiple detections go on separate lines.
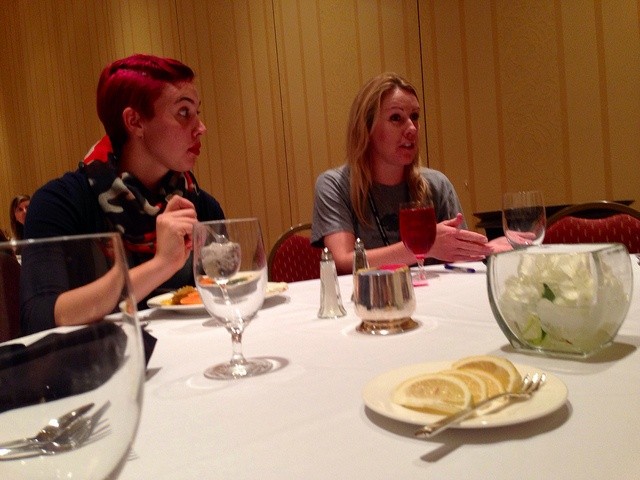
165, 191, 229, 244
413, 369, 547, 441
1, 417, 114, 461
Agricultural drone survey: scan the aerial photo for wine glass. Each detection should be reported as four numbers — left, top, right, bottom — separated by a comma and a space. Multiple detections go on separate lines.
1, 233, 148, 480
398, 200, 441, 280
191, 216, 273, 380
202, 239, 242, 306
501, 188, 545, 250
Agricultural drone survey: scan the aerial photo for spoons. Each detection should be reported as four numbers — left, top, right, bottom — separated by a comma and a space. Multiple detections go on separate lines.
1, 402, 93, 452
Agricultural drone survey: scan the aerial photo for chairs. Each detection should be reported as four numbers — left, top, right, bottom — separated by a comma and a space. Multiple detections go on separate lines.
0, 232, 22, 340
268, 223, 325, 280
535, 201, 640, 255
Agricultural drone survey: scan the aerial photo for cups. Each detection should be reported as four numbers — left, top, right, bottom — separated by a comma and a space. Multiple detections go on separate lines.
483, 242, 634, 358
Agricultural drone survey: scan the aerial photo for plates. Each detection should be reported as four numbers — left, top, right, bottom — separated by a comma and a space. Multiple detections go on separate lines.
361, 360, 569, 430
144, 280, 290, 316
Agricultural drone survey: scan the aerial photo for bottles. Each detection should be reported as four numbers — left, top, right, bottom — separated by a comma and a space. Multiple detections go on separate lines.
315, 246, 348, 319
347, 238, 371, 299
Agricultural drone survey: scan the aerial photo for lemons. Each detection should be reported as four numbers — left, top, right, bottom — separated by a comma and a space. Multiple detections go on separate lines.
438, 370, 487, 406
454, 355, 523, 393
459, 369, 505, 399
392, 374, 471, 414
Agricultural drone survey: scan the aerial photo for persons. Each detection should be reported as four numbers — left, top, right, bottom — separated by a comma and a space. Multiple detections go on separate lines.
310, 72, 535, 277
18, 55, 229, 335
10, 193, 31, 242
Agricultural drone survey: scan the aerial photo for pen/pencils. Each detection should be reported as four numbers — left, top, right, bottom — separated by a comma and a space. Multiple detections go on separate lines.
444, 264, 476, 273
